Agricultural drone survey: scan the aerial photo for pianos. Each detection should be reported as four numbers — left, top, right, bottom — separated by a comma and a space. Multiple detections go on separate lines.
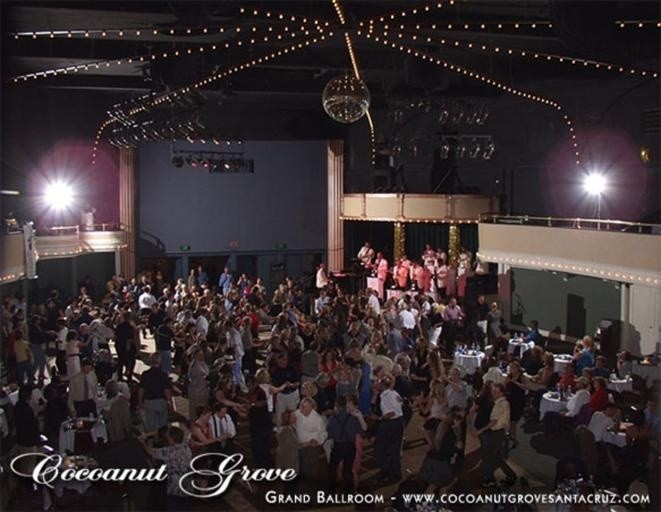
331, 271, 367, 294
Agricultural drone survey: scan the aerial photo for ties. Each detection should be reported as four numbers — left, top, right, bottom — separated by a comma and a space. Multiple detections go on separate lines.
219, 420, 226, 448
84, 374, 89, 401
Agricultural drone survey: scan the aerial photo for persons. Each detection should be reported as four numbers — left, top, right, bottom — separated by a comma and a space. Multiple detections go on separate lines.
79, 201, 90, 225
83, 207, 98, 232
24, 215, 35, 226
1, 239, 661, 512
38, 223, 50, 236
3, 209, 19, 228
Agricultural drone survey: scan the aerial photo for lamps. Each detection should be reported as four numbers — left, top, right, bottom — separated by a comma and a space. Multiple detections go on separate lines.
320, 19, 372, 127
170, 145, 232, 174
